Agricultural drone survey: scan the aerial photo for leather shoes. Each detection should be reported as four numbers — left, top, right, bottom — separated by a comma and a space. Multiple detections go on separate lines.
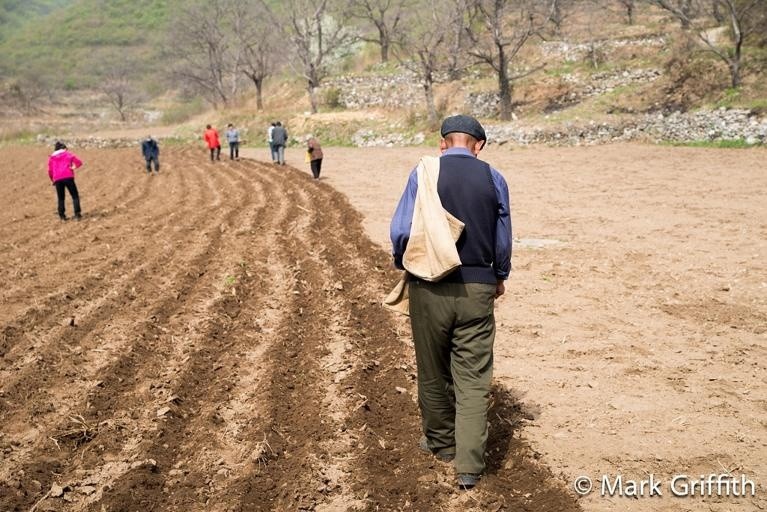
457, 473, 480, 488
420, 435, 453, 462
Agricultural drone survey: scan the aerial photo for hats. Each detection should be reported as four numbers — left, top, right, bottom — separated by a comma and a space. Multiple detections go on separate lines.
441, 114, 486, 150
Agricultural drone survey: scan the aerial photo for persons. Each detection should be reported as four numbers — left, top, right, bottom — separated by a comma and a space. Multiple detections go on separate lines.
204, 124, 222, 162
267, 121, 288, 166
389, 116, 512, 488
225, 123, 241, 161
48, 141, 83, 222
141, 134, 160, 176
306, 132, 324, 181
